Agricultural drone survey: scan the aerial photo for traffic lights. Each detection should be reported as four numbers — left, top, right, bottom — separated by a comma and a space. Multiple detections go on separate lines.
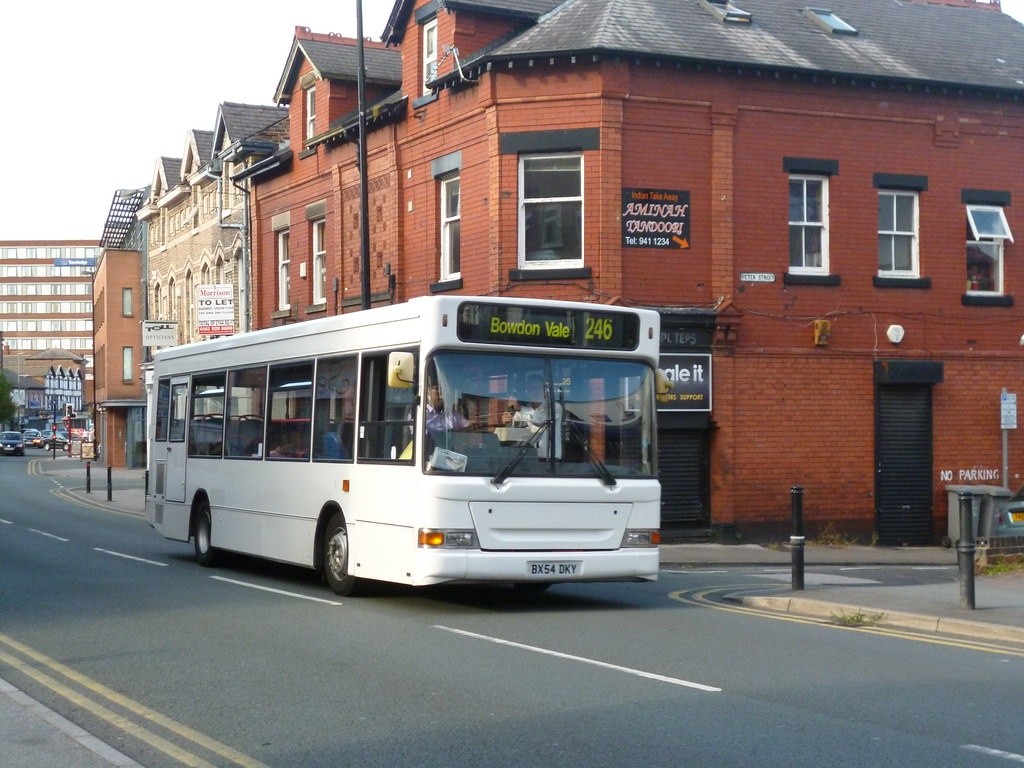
67, 405, 72, 417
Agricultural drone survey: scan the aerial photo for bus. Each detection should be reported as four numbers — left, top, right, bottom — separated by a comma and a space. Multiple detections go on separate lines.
144, 295, 661, 597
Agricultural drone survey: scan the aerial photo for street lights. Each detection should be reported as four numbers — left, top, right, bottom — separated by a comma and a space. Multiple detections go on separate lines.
53, 395, 56, 460
18, 353, 24, 431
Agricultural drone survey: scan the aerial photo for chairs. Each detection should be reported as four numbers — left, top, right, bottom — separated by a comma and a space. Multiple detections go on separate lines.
188, 414, 361, 460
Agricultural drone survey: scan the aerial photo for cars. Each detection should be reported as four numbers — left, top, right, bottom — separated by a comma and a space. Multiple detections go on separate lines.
43, 432, 83, 451
996, 483, 1024, 534
0, 431, 25, 456
41, 430, 52, 447
19, 429, 44, 449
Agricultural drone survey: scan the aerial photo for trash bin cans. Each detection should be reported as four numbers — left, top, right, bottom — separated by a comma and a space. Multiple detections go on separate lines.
942, 484, 1014, 549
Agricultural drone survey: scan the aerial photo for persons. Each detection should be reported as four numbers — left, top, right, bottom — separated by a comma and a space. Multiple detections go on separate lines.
271, 430, 310, 458
502, 374, 563, 463
405, 384, 480, 461
